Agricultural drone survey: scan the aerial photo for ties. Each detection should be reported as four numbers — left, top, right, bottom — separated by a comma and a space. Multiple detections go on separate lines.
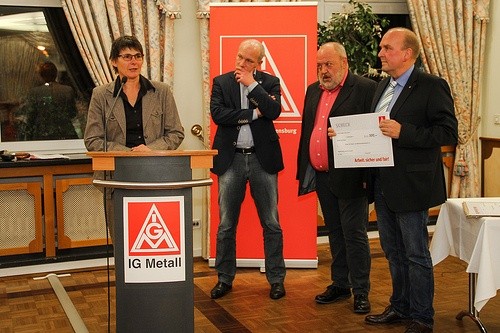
378, 81, 398, 114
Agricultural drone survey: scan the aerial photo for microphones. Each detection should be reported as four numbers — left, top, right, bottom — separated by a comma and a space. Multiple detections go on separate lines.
104, 76, 128, 152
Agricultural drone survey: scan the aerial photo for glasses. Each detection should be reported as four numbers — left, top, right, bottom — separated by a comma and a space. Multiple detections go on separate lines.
118, 53, 145, 60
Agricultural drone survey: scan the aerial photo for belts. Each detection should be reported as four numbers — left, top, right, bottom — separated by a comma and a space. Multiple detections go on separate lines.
235, 147, 255, 153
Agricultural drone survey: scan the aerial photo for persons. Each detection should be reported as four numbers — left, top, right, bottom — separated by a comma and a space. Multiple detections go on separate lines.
28, 62, 78, 141
326, 26, 458, 333
84, 35, 186, 244
296, 42, 378, 314
210, 39, 287, 300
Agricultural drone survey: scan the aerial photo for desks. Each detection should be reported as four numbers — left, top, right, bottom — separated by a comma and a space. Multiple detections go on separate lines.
0, 148, 116, 278
426, 196, 500, 332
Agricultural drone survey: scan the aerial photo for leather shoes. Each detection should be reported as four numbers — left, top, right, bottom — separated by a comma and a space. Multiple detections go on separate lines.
316, 286, 351, 304
270, 282, 285, 300
211, 282, 232, 298
365, 306, 410, 326
355, 295, 370, 313
405, 322, 432, 333
